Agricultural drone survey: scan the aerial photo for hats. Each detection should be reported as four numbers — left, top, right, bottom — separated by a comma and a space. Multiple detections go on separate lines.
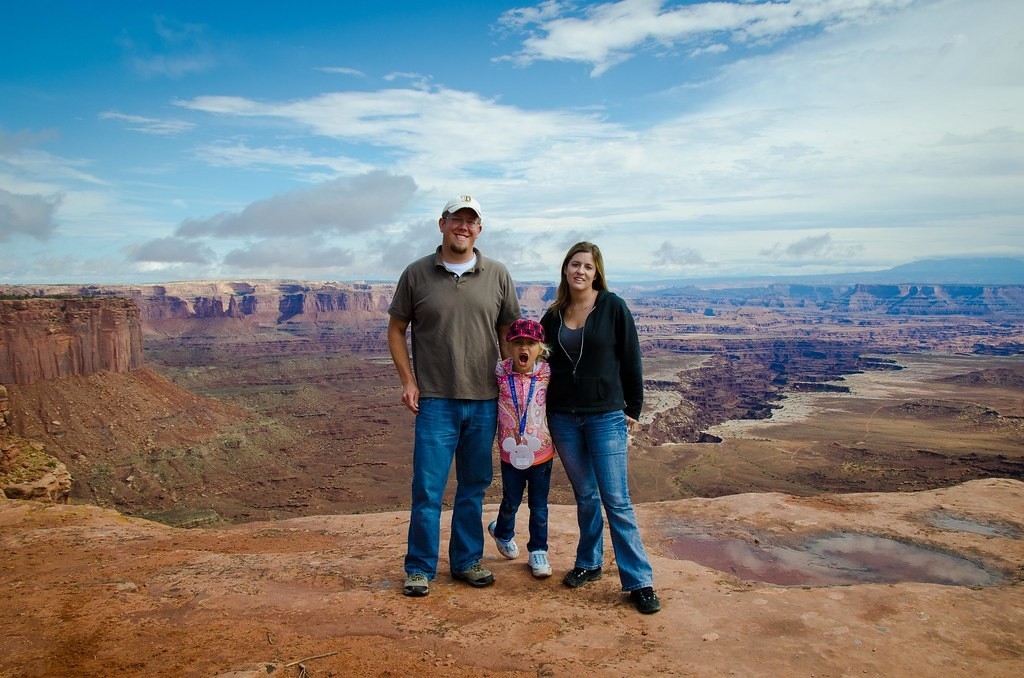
503, 316, 544, 343
441, 195, 483, 220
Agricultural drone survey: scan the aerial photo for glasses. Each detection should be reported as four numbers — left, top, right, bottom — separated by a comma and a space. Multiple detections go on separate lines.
443, 216, 480, 225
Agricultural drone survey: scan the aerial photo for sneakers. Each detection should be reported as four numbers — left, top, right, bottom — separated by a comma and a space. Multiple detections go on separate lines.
489, 519, 520, 559
525, 549, 552, 577
628, 589, 664, 614
563, 567, 601, 586
448, 564, 494, 586
402, 570, 430, 596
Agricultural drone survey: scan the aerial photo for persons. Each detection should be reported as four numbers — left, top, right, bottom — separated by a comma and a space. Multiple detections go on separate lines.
487, 322, 555, 579
538, 241, 661, 615
388, 194, 521, 597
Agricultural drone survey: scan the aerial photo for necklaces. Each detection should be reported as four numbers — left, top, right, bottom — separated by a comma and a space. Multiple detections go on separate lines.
568, 289, 594, 311
